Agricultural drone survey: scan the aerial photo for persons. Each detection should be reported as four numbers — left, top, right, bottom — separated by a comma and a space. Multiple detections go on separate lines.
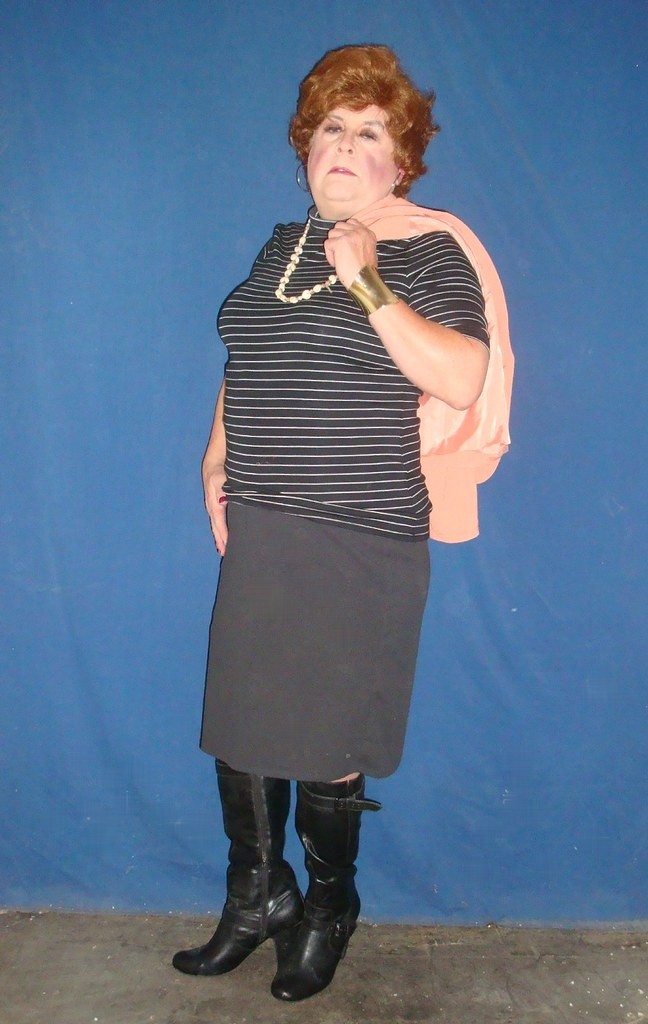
172, 46, 515, 1001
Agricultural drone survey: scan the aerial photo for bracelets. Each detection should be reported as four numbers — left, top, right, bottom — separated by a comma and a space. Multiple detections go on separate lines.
347, 264, 399, 316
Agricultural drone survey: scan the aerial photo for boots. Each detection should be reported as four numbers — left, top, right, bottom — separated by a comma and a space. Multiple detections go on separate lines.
172, 759, 305, 976
270, 769, 382, 1002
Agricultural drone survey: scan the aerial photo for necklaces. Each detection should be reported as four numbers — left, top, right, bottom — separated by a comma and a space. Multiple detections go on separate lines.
275, 219, 337, 303
